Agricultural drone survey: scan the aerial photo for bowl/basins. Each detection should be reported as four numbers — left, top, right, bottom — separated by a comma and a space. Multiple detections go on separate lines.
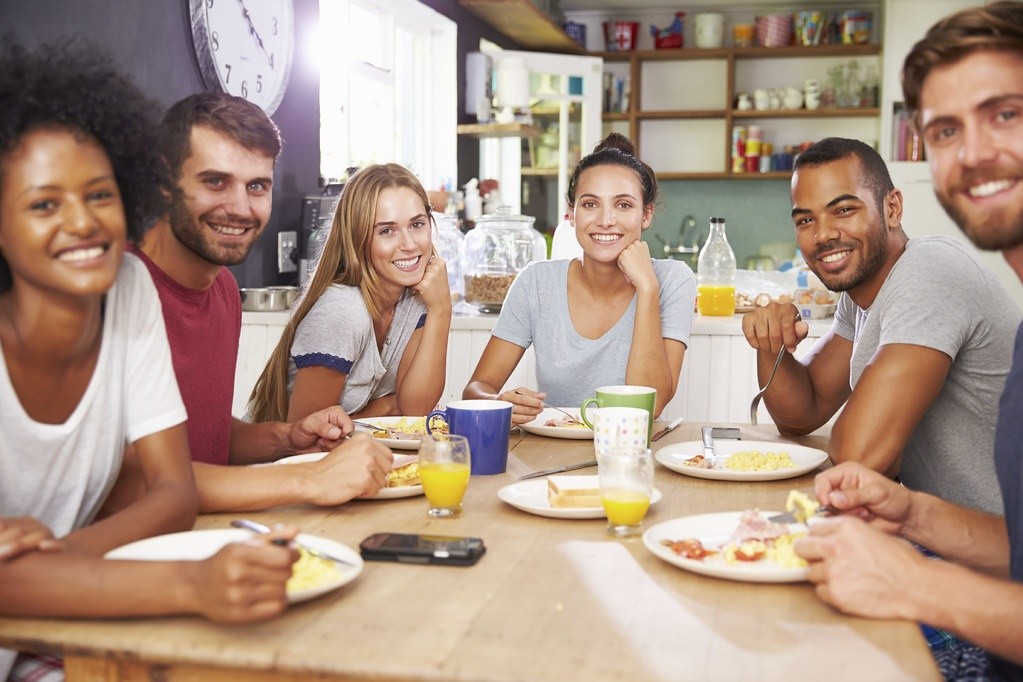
238, 285, 300, 312
739, 77, 818, 111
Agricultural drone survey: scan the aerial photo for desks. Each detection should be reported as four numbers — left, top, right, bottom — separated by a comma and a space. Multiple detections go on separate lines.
0, 419, 945, 682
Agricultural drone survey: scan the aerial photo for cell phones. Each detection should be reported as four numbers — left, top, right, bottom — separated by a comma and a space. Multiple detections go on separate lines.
710, 428, 741, 440
359, 533, 486, 567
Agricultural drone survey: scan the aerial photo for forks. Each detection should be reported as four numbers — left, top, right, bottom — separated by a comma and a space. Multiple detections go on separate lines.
751, 314, 802, 427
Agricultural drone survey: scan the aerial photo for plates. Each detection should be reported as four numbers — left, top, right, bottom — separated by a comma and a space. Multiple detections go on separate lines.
273, 452, 424, 499
102, 526, 363, 605
346, 416, 426, 451
517, 407, 594, 440
643, 511, 834, 582
655, 439, 828, 482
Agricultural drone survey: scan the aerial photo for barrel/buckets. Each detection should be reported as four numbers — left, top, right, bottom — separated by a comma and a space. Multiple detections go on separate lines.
570, 11, 878, 53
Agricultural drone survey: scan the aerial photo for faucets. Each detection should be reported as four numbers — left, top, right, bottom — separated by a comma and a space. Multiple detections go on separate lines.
677, 215, 696, 247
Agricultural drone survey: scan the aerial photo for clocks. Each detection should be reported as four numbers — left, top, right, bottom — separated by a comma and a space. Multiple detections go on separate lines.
188, 0, 295, 118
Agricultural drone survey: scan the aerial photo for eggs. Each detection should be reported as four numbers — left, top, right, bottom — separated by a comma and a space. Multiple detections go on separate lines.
799, 293, 834, 304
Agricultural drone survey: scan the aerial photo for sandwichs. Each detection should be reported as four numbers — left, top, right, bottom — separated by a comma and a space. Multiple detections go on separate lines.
386, 455, 421, 486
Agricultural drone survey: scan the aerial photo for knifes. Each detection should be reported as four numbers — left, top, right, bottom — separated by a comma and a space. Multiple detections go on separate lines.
701, 426, 717, 467
230, 519, 355, 566
769, 504, 834, 523
518, 461, 597, 476
653, 415, 683, 442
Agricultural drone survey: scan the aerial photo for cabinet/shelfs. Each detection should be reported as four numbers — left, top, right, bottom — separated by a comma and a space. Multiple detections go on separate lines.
458, 0, 883, 179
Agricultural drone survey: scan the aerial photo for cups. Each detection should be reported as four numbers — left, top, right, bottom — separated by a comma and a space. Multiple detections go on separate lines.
425, 398, 513, 474
597, 447, 655, 534
590, 407, 649, 472
417, 434, 471, 516
729, 126, 808, 174
581, 385, 657, 448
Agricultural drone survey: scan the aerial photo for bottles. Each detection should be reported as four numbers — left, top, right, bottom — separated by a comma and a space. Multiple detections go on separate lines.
433, 206, 585, 314
696, 214, 739, 317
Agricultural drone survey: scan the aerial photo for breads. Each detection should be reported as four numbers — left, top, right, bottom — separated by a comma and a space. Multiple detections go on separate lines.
548, 475, 599, 508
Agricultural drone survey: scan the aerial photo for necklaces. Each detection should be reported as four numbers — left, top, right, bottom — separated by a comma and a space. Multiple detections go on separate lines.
373, 308, 394, 345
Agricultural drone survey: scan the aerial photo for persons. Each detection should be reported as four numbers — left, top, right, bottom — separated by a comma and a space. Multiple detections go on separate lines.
126, 91, 393, 513
461, 132, 697, 431
793, 0, 1022, 665
1, 28, 302, 682
742, 137, 1022, 682
237, 163, 452, 425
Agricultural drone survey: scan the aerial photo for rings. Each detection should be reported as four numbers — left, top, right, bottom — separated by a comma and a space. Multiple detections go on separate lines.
514, 405, 517, 413
